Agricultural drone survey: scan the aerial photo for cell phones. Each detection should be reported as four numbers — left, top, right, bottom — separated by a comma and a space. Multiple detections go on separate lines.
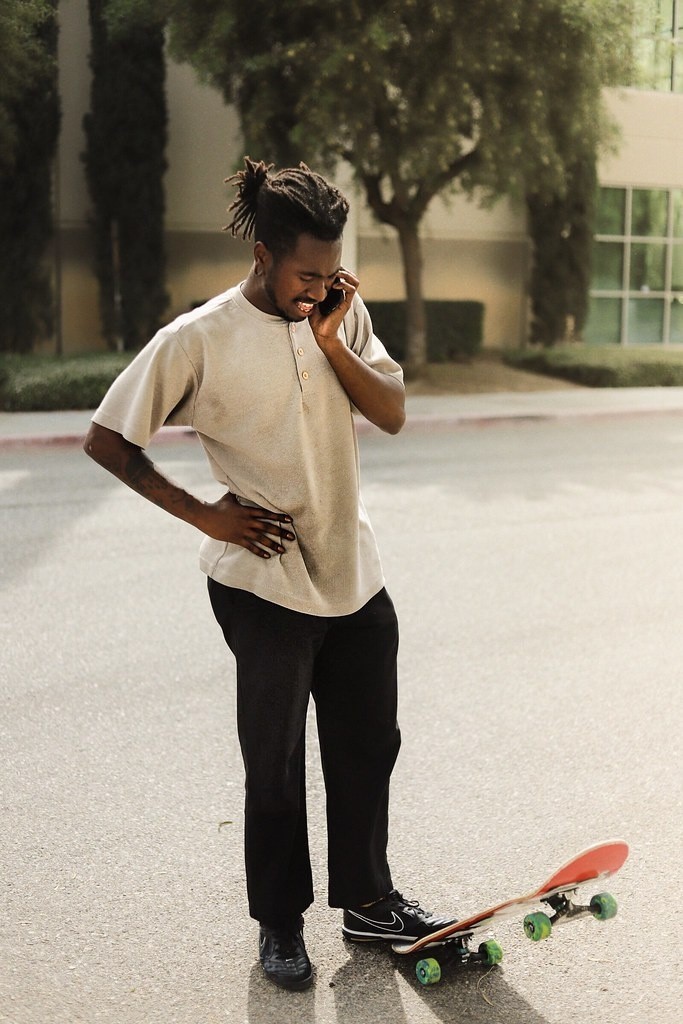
318, 277, 345, 316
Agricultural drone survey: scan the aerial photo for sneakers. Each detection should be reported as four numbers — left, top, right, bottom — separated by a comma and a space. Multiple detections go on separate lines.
342, 890, 458, 944
259, 917, 313, 989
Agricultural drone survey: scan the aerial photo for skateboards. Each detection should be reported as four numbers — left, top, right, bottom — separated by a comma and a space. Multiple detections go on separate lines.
391, 841, 630, 983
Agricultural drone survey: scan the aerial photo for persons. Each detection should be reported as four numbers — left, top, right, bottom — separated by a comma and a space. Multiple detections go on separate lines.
82, 157, 459, 991
